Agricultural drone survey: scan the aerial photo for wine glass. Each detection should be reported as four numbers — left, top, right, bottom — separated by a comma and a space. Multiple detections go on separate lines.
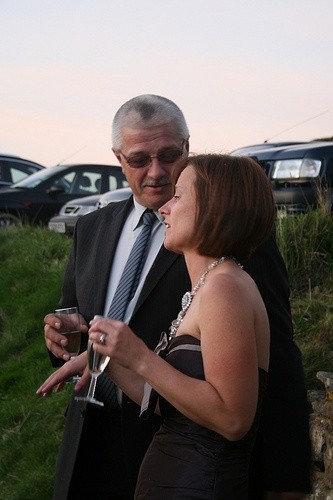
56, 307, 81, 383
75, 315, 110, 407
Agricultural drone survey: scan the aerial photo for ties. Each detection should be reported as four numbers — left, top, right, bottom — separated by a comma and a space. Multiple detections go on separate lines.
95, 209, 157, 410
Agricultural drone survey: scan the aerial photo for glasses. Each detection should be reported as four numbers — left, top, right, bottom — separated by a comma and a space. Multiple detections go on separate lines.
120, 140, 184, 168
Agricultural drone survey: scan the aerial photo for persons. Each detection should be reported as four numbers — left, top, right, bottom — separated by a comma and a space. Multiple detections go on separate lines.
42, 94, 315, 500
35, 154, 277, 500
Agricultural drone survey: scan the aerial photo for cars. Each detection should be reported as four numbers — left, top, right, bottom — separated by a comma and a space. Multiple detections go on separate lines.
47, 138, 333, 235
0, 164, 130, 230
1, 153, 92, 193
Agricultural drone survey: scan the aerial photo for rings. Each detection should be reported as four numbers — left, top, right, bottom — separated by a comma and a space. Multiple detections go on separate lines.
99, 335, 105, 344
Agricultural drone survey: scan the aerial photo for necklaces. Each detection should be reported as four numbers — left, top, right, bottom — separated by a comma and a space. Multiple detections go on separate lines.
169, 254, 244, 336
